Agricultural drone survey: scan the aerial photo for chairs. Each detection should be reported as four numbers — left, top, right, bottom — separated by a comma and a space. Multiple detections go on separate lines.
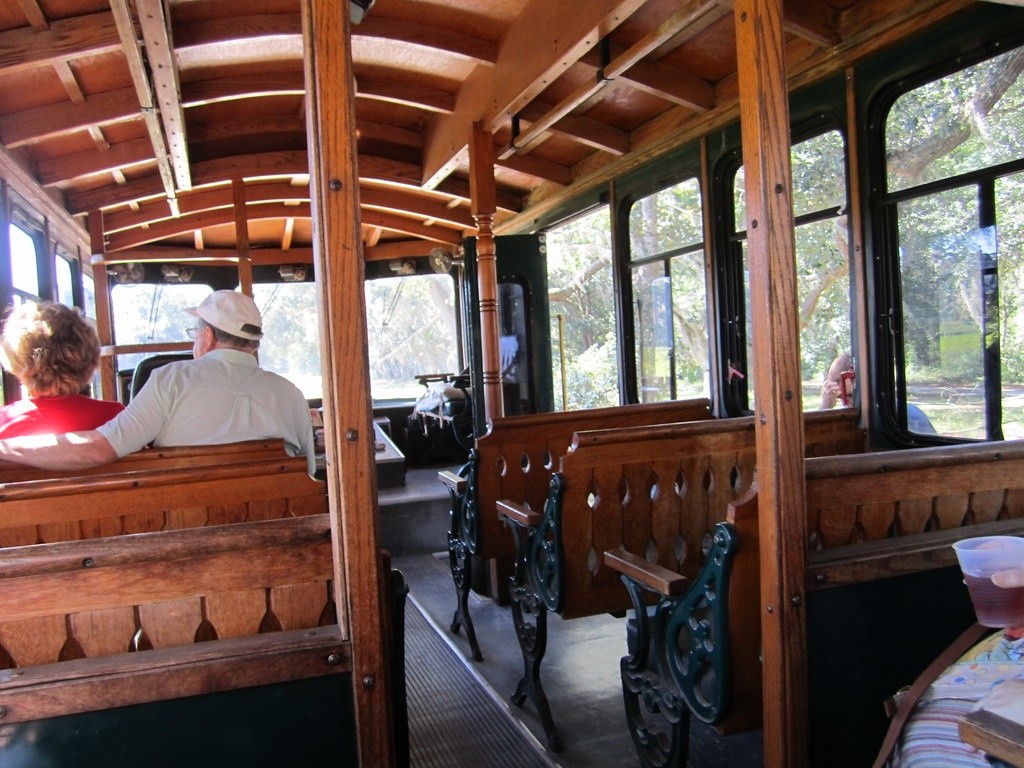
410, 334, 521, 455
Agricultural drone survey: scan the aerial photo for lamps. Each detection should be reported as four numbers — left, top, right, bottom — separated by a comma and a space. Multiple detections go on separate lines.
350, 0, 375, 25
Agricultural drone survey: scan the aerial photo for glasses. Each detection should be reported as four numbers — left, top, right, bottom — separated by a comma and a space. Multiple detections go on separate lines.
186, 328, 198, 339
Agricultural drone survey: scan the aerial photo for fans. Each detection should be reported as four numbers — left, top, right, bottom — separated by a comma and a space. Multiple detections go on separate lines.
428, 247, 465, 274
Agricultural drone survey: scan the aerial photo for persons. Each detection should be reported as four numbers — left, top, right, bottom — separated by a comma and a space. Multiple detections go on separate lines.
0, 299, 127, 439
818, 352, 939, 436
0, 289, 316, 477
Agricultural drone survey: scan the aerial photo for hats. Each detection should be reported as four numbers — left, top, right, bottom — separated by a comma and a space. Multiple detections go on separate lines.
181, 289, 263, 340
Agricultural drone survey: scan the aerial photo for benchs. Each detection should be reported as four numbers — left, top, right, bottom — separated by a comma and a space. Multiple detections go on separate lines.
0, 438, 411, 768
432, 396, 712, 666
603, 440, 1024, 768
491, 405, 867, 751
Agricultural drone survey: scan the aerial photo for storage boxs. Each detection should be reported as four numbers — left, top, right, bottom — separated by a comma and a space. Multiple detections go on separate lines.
106, 262, 144, 287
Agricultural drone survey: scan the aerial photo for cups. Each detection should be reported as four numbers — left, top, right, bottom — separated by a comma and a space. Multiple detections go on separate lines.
951, 536, 1024, 627
